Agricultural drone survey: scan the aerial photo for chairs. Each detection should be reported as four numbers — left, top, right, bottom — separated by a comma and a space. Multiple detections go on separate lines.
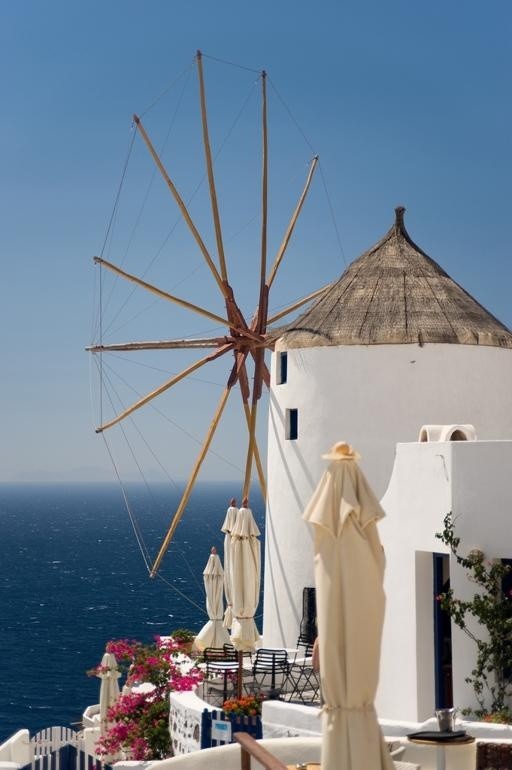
194, 634, 320, 709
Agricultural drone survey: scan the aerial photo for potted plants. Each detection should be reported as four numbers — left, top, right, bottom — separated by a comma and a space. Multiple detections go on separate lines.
171, 629, 195, 654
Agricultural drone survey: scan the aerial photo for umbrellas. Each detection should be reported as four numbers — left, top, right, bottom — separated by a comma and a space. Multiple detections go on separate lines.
218, 498, 243, 627
300, 443, 397, 770
96, 653, 122, 737
228, 496, 266, 655
194, 545, 234, 659
